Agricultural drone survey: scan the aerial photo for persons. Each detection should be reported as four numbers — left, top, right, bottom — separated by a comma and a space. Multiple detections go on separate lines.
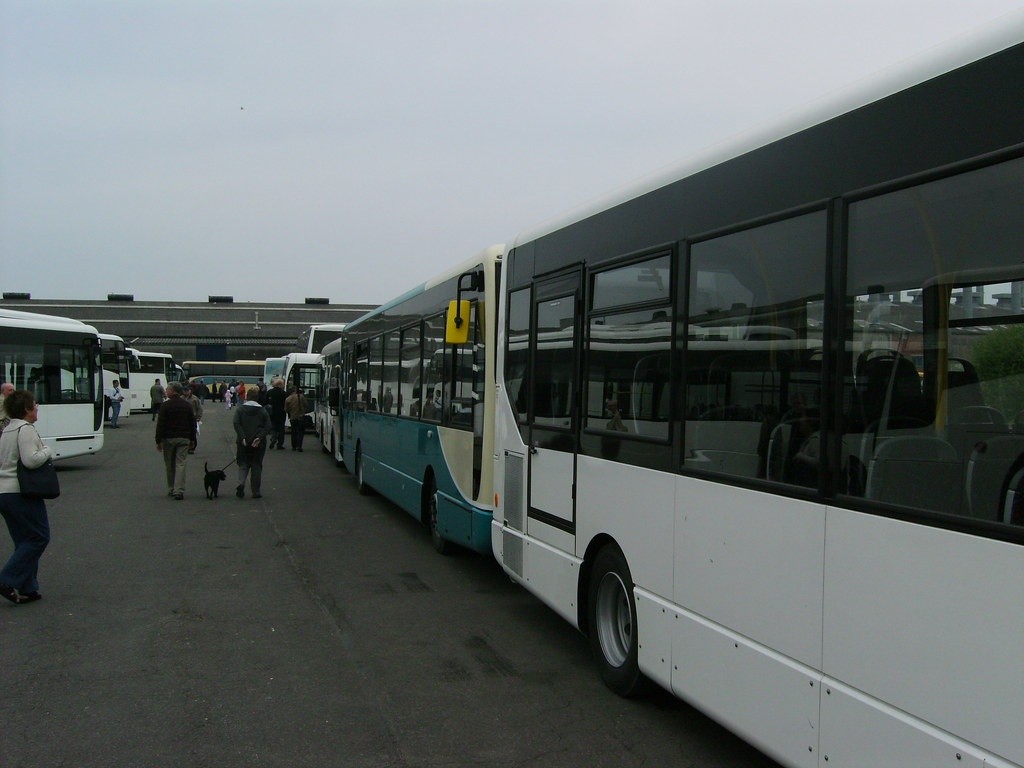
224, 390, 233, 409
266, 379, 290, 449
109, 380, 124, 429
155, 381, 197, 499
219, 380, 227, 402
212, 381, 217, 403
150, 379, 167, 421
0, 383, 51, 603
234, 389, 272, 499
285, 385, 310, 452
228, 379, 245, 406
182, 386, 203, 453
785, 393, 820, 485
258, 384, 269, 407
184, 379, 208, 405
423, 391, 436, 419
383, 388, 393, 413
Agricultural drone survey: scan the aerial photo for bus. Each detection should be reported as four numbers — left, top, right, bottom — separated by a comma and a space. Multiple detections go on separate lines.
489, 39, 1024, 767
338, 243, 510, 559
0, 308, 107, 462
98, 321, 346, 467
504, 318, 983, 493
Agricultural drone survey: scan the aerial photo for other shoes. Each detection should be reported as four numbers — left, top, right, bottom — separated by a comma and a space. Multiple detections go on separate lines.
252, 492, 263, 498
292, 446, 297, 451
19, 591, 41, 602
236, 485, 244, 498
0, 583, 29, 604
175, 492, 184, 500
188, 450, 195, 454
111, 424, 120, 430
269, 440, 277, 449
296, 446, 303, 452
277, 443, 285, 449
169, 489, 175, 496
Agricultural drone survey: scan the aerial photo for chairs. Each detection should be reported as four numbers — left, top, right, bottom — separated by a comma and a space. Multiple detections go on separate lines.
857, 417, 938, 494
705, 352, 789, 422
792, 346, 824, 412
855, 348, 905, 401
948, 358, 990, 423
966, 436, 1024, 523
865, 436, 963, 516
632, 352, 708, 438
948, 405, 1008, 465
695, 405, 766, 473
766, 417, 850, 491
860, 356, 923, 429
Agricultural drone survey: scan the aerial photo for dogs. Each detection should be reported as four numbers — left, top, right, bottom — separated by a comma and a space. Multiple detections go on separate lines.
203, 461, 227, 500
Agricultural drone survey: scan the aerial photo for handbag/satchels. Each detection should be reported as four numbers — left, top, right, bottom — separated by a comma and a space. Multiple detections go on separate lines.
16, 423, 61, 500
298, 415, 313, 428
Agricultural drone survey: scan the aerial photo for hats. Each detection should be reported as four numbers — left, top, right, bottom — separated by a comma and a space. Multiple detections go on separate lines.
182, 382, 193, 390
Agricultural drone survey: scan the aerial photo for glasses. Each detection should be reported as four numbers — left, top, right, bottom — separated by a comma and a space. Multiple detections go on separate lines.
31, 402, 39, 407
